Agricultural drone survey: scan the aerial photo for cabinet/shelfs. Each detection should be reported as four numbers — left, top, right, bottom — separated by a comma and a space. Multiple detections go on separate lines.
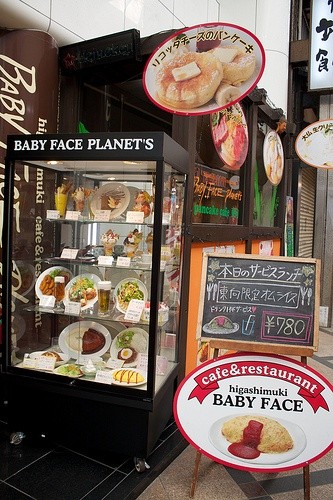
2, 130, 191, 412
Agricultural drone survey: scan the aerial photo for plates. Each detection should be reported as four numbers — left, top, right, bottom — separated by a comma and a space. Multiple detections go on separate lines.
90, 182, 130, 219
110, 368, 147, 387
84, 319, 127, 352
120, 186, 141, 218
208, 412, 307, 466
58, 320, 112, 360
110, 327, 149, 368
62, 273, 101, 310
31, 351, 70, 366
52, 363, 84, 378
112, 278, 148, 314
35, 266, 73, 303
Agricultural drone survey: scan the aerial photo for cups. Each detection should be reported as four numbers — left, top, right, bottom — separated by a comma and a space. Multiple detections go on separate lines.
54, 188, 68, 219
96, 281, 117, 318
101, 240, 152, 259
73, 191, 85, 216
133, 192, 151, 219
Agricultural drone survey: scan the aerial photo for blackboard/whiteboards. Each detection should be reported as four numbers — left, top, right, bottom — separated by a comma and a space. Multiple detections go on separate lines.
196, 250, 322, 355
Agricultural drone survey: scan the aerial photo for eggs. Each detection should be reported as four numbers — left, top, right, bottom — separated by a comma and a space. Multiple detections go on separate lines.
130, 332, 147, 352
121, 348, 132, 359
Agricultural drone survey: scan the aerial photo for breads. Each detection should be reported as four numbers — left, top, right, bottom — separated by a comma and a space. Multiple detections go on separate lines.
154, 52, 222, 108
204, 45, 255, 84
112, 369, 144, 383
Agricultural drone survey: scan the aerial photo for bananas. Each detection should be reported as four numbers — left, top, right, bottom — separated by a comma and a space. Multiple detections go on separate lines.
171, 62, 202, 82
215, 83, 242, 108
211, 48, 239, 64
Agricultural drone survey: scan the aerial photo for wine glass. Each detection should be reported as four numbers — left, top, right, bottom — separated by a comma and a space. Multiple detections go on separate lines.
54, 276, 65, 311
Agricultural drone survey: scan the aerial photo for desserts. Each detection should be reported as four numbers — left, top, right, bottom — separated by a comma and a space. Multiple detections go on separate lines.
220, 415, 294, 458
64, 327, 106, 355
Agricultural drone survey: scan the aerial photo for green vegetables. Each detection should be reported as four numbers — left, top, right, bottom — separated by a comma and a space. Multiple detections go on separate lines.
116, 330, 134, 348
57, 365, 79, 375
117, 281, 143, 303
50, 270, 69, 279
71, 276, 94, 301
212, 106, 240, 127
